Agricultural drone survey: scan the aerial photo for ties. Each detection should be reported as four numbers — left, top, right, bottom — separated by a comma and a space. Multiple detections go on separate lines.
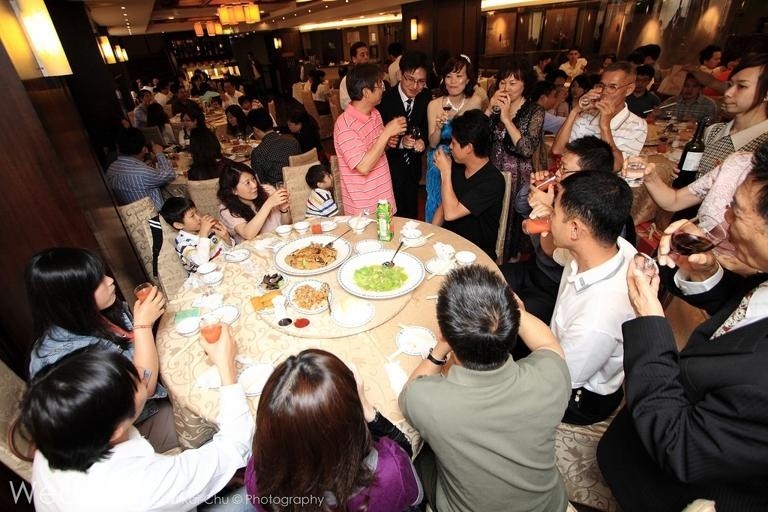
405, 98, 413, 122
708, 278, 768, 338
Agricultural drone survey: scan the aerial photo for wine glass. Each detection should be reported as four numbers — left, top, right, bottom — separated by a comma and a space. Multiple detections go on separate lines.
440, 96, 453, 125
411, 128, 421, 153
634, 214, 728, 277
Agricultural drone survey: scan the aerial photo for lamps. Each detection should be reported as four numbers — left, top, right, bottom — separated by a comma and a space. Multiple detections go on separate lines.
95, 34, 130, 66
0, 1, 74, 82
192, 1, 262, 38
273, 37, 280, 50
410, 17, 418, 42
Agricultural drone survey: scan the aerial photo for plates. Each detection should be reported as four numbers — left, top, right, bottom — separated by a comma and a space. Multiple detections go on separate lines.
255, 215, 476, 360
237, 364, 278, 395
150, 108, 254, 176
175, 249, 250, 337
644, 119, 693, 148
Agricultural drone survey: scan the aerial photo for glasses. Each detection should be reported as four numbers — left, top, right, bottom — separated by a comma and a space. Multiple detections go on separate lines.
554, 159, 581, 178
591, 80, 634, 96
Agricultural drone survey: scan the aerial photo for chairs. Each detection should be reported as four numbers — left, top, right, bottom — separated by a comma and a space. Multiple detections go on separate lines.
1, 83, 716, 511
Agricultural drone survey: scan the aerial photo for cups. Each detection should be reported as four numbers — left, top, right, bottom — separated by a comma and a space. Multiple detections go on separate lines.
134, 283, 157, 303
200, 316, 222, 355
656, 141, 667, 153
491, 92, 510, 114
625, 155, 649, 188
534, 172, 558, 191
394, 116, 406, 136
347, 364, 359, 382
276, 182, 289, 202
581, 93, 605, 108
433, 148, 453, 163
201, 213, 219, 229
522, 215, 552, 235
559, 89, 569, 97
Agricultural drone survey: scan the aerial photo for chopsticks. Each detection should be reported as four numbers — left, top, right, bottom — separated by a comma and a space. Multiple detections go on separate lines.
354, 212, 362, 233
644, 102, 677, 113
242, 350, 285, 392
169, 333, 202, 361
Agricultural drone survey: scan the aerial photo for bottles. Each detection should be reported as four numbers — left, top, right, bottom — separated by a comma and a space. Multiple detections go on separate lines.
667, 112, 672, 122
671, 118, 708, 189
